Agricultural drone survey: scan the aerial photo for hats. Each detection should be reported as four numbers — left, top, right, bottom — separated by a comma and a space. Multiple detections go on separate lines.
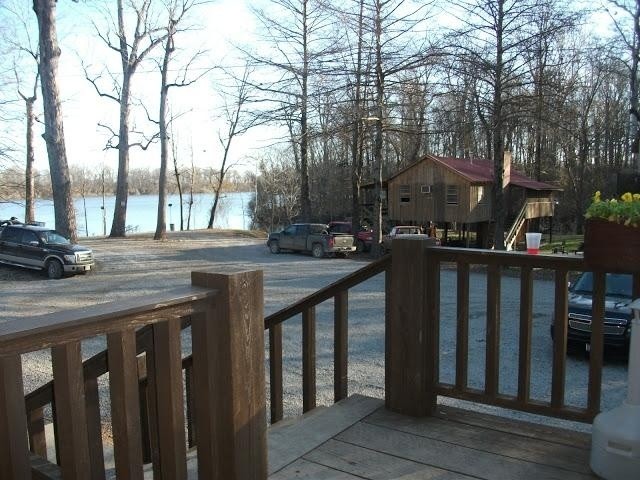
11, 217, 18, 220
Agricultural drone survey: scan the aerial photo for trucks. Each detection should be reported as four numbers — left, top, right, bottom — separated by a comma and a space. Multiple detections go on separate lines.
327, 221, 374, 253
378, 224, 441, 256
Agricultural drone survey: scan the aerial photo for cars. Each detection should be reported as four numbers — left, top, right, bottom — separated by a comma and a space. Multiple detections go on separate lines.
267, 220, 357, 259
550, 272, 633, 356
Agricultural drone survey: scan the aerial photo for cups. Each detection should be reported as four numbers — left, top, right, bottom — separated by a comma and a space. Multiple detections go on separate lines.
524, 232, 543, 254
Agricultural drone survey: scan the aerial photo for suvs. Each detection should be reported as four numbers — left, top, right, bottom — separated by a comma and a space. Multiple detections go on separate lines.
0, 221, 96, 280
